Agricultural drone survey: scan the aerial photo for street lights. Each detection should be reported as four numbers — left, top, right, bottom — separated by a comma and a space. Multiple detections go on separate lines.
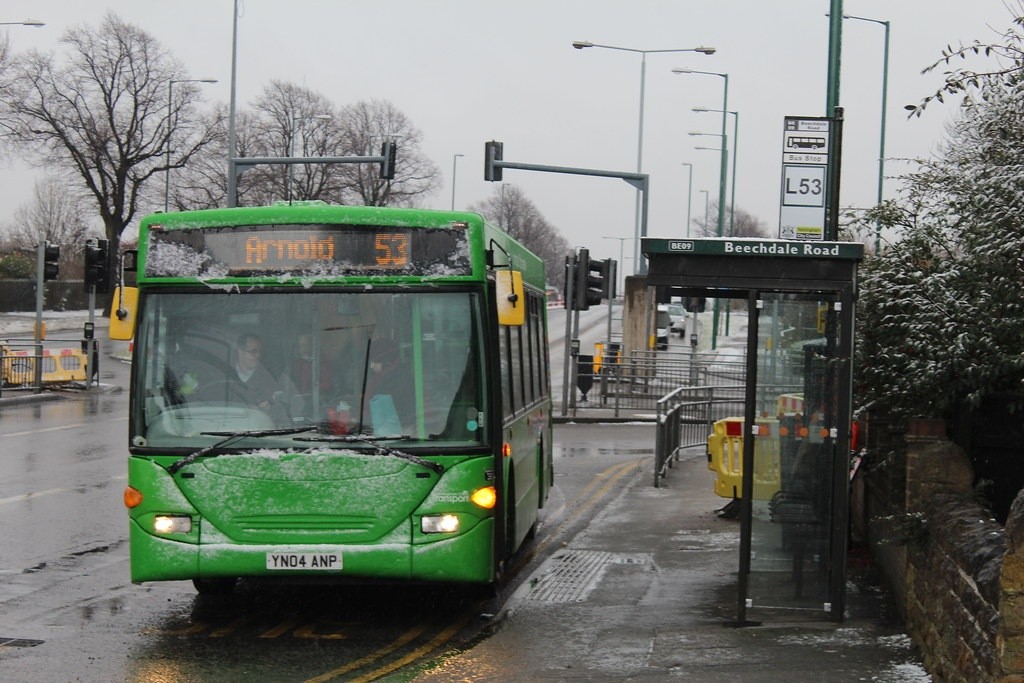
823, 12, 891, 256
691, 108, 739, 337
164, 79, 219, 212
602, 235, 635, 301
671, 68, 728, 350
288, 114, 331, 205
500, 183, 511, 228
689, 131, 729, 336
681, 164, 709, 241
573, 41, 717, 273
451, 154, 465, 210
369, 134, 402, 206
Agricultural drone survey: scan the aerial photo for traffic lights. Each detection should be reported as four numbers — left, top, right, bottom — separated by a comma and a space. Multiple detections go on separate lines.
563, 248, 617, 310
84, 237, 108, 291
44, 241, 60, 280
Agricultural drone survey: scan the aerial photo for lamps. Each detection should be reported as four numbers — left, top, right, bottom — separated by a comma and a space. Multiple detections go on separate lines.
691, 334, 698, 344
83, 322, 94, 337
570, 339, 580, 349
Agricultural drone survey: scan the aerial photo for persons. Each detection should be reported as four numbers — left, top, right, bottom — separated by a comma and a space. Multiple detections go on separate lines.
220, 332, 272, 411
290, 332, 417, 428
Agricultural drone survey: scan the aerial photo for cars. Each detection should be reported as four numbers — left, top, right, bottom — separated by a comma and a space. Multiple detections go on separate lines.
658, 304, 689, 339
656, 306, 674, 350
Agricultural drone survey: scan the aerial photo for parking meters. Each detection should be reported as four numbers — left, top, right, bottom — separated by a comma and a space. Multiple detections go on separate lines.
569, 339, 580, 407
84, 321, 95, 387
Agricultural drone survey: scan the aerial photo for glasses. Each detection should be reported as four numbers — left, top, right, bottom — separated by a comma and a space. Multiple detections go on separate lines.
244, 348, 264, 356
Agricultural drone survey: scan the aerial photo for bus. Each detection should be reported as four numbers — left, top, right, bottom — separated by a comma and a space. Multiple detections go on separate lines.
109, 203, 554, 598
